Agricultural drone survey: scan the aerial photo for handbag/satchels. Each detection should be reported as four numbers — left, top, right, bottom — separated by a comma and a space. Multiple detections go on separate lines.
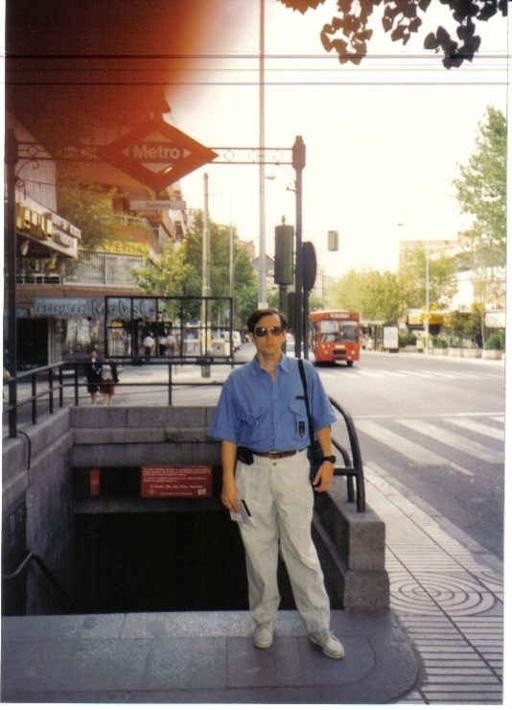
234, 445, 254, 466
308, 439, 329, 482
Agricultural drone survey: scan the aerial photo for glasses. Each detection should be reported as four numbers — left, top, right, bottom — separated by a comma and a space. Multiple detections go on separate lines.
253, 325, 284, 337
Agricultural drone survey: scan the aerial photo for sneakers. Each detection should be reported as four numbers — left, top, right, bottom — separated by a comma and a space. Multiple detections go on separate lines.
307, 628, 347, 660
251, 614, 278, 650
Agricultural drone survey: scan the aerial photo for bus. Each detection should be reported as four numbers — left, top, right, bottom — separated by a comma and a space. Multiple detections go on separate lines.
308, 309, 361, 367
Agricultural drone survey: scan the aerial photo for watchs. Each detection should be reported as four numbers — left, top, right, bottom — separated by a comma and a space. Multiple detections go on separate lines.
321, 455, 336, 464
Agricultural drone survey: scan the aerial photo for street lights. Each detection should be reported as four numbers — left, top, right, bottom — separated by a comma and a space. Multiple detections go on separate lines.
228, 223, 254, 325
424, 247, 440, 350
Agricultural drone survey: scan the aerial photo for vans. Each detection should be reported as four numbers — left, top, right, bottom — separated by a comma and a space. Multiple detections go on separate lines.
221, 330, 242, 350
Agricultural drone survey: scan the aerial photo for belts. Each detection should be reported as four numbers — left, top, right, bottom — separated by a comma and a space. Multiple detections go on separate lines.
250, 446, 307, 460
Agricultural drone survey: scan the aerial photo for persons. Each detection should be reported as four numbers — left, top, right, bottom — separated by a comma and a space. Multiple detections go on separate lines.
100, 354, 116, 404
362, 336, 373, 352
88, 349, 98, 405
206, 308, 344, 659
167, 331, 176, 357
157, 331, 167, 361
143, 331, 156, 362
